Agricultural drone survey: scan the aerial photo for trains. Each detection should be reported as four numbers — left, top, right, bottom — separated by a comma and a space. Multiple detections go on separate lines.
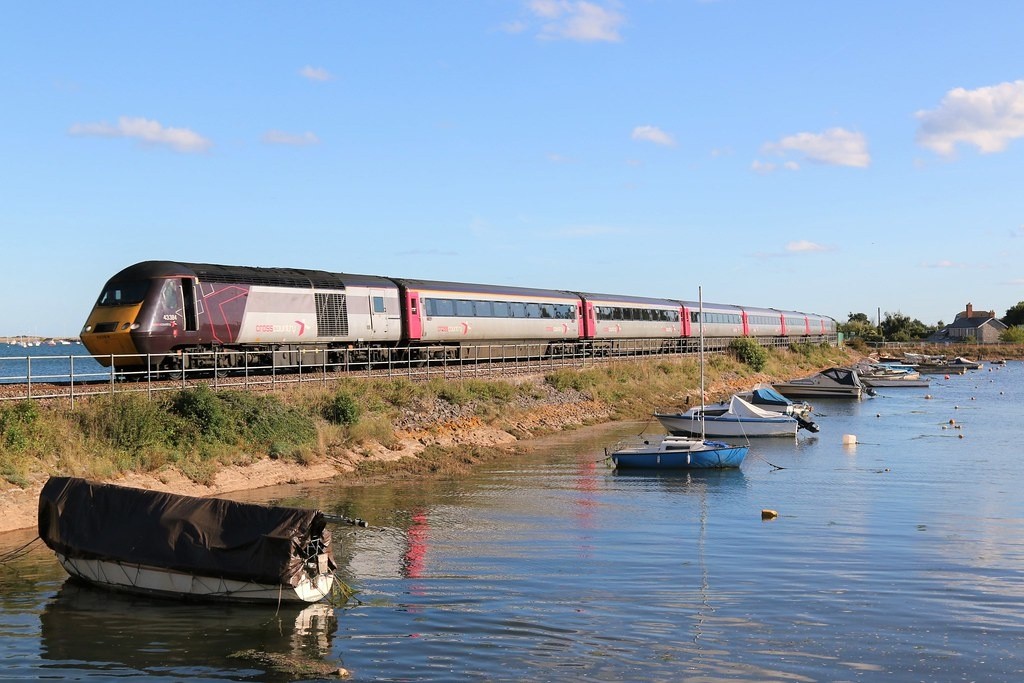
79, 259, 839, 382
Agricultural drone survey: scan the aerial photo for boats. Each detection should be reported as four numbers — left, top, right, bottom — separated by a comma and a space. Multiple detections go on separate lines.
845, 351, 1007, 388
768, 366, 877, 398
653, 394, 821, 438
696, 382, 814, 420
36, 473, 369, 603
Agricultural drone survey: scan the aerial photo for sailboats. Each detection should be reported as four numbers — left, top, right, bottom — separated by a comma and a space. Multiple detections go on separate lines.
603, 286, 752, 470
